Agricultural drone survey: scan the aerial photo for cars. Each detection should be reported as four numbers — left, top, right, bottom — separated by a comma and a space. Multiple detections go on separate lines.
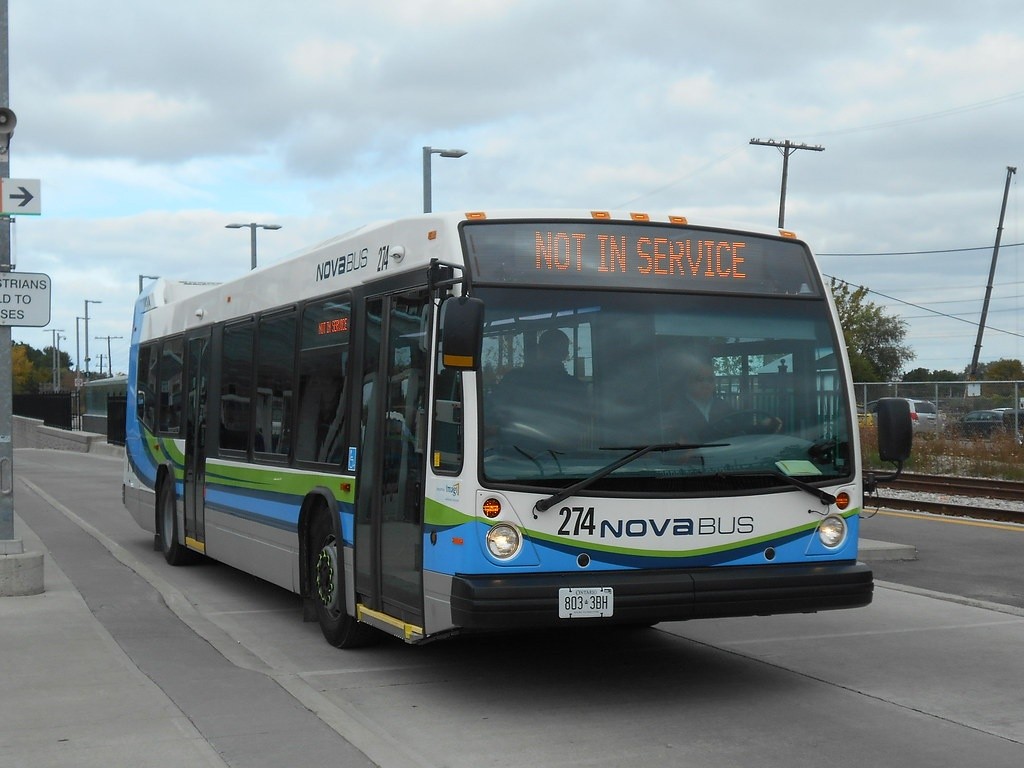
854, 396, 1024, 441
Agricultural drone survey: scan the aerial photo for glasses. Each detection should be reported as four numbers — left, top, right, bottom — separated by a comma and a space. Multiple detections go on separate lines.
697, 375, 716, 384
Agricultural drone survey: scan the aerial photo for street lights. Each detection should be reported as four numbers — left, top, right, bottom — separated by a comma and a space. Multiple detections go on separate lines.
76, 316, 91, 382
84, 300, 102, 382
422, 146, 468, 214
139, 275, 160, 294
225, 222, 282, 271
57, 333, 66, 392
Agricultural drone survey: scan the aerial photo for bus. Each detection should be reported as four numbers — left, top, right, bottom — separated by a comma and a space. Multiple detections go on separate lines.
123, 204, 913, 650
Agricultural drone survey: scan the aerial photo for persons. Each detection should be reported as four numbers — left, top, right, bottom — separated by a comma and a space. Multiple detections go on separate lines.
667, 360, 783, 442
489, 329, 589, 460
